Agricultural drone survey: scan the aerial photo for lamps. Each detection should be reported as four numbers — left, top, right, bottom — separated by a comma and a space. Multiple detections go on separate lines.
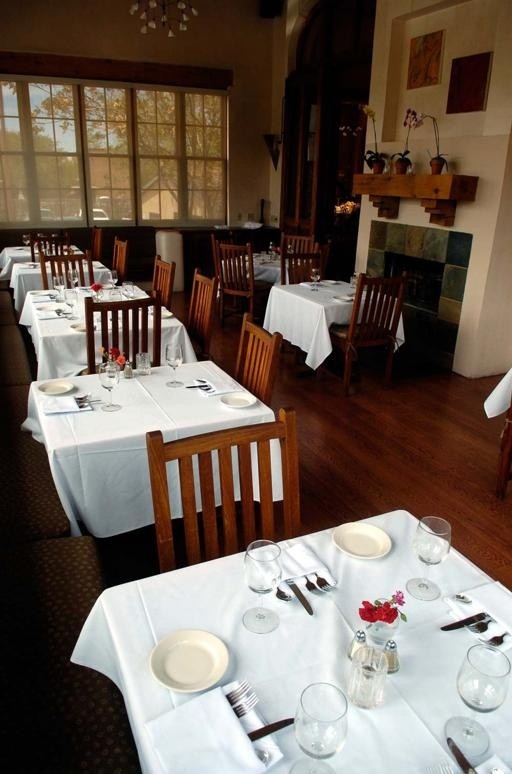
127, 0, 198, 37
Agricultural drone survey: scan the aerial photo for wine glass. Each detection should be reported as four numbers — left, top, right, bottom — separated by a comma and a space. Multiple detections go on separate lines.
164, 343, 186, 385
20, 230, 67, 254
243, 539, 284, 634
250, 240, 367, 303
24, 269, 174, 332
406, 515, 451, 603
443, 644, 511, 757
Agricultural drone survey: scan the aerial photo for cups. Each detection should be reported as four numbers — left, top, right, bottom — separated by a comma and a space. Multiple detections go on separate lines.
346, 646, 390, 710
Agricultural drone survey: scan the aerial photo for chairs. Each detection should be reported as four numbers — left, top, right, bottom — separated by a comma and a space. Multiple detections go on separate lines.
1, 228, 303, 571
210, 232, 406, 398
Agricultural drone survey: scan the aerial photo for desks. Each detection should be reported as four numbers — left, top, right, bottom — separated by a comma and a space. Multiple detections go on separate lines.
494, 368, 512, 500
102, 509, 511, 774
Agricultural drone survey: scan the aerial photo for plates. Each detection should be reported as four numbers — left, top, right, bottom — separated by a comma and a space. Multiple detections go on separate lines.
150, 630, 229, 693
35, 352, 258, 411
331, 523, 393, 561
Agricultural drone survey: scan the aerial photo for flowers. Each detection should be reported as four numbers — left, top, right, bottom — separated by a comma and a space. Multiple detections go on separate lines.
416, 112, 444, 159
394, 108, 417, 168
360, 105, 388, 166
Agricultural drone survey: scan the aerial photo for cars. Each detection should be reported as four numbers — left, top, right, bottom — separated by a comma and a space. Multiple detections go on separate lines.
20, 185, 123, 221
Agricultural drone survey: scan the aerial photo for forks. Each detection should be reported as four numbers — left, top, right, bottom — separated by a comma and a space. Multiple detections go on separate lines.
479, 631, 509, 647
232, 694, 258, 718
464, 619, 495, 634
303, 572, 337, 597
224, 679, 251, 706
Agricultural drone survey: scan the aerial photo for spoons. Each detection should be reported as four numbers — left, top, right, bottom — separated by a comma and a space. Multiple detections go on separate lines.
254, 746, 269, 766
452, 595, 472, 604
288, 681, 349, 773
274, 586, 293, 602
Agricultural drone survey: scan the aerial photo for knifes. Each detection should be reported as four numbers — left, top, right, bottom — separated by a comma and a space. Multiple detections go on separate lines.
440, 611, 490, 631
446, 735, 479, 774
286, 579, 314, 616
247, 716, 295, 742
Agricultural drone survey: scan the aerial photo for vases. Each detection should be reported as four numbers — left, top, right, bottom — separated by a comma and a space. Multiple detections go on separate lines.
392, 162, 408, 175
372, 164, 385, 174
430, 161, 445, 174
